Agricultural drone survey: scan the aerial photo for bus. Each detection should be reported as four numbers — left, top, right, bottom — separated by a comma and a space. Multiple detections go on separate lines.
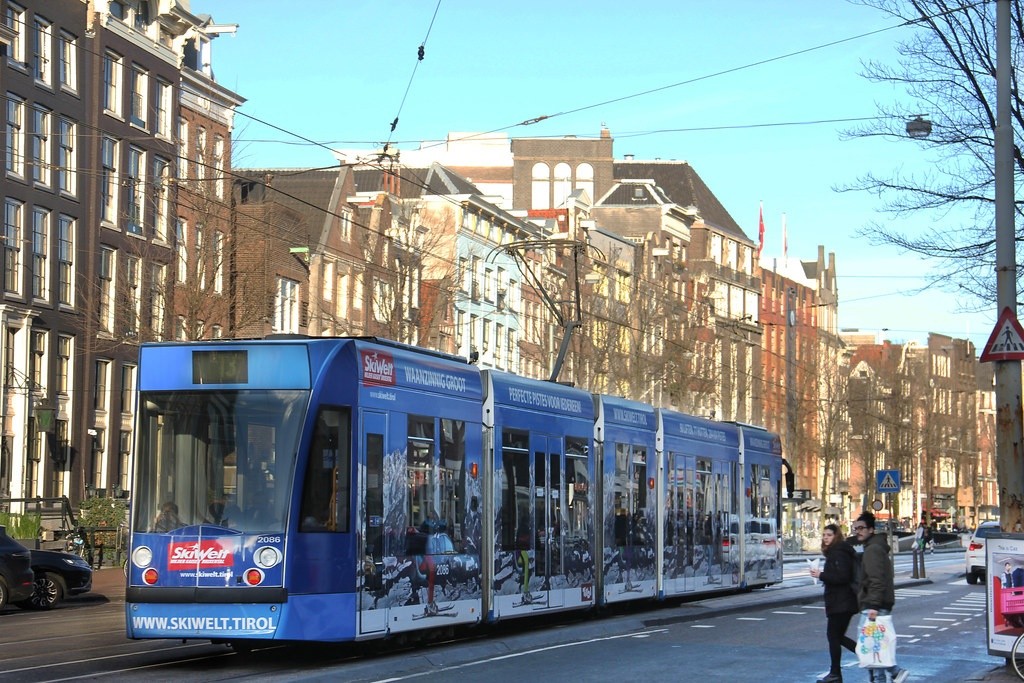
125, 240, 796, 654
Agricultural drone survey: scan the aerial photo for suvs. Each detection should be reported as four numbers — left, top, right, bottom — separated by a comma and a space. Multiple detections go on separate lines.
874, 519, 905, 536
965, 519, 1001, 584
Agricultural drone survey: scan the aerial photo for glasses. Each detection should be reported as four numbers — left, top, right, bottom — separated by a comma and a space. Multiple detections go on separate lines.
852, 526, 870, 532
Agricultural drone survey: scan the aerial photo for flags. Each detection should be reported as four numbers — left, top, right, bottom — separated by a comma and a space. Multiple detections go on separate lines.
783, 219, 788, 268
759, 206, 765, 252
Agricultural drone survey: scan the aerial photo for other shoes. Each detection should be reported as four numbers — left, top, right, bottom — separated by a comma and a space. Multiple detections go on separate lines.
817, 673, 842, 683
891, 669, 909, 683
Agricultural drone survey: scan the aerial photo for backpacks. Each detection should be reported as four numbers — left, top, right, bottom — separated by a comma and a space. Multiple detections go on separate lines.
830, 546, 863, 595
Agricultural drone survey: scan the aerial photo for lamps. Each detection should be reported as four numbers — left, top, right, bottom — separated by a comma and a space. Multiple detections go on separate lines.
907, 116, 931, 138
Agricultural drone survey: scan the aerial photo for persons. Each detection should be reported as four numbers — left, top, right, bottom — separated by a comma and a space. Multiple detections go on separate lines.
621, 548, 634, 591
616, 513, 638, 548
914, 521, 934, 554
810, 524, 865, 683
421, 511, 454, 538
149, 502, 188, 531
855, 511, 910, 683
419, 556, 438, 615
952, 523, 961, 532
517, 550, 533, 603
213, 473, 276, 527
941, 525, 947, 532
1001, 562, 1015, 626
704, 510, 723, 564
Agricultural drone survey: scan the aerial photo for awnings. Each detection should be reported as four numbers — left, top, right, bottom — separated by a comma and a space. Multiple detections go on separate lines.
924, 509, 949, 516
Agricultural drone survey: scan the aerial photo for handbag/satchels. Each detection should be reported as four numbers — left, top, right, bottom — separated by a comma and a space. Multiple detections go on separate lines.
911, 538, 919, 550
855, 615, 897, 668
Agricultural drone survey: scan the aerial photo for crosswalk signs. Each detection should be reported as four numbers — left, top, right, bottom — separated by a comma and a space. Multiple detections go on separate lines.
876, 470, 902, 493
980, 306, 1024, 363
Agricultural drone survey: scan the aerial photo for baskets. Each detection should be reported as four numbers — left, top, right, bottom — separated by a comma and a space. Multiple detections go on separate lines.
1000, 587, 1024, 628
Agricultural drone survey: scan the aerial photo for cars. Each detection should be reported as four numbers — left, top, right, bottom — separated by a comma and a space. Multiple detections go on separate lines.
369, 531, 454, 556
0, 525, 93, 611
